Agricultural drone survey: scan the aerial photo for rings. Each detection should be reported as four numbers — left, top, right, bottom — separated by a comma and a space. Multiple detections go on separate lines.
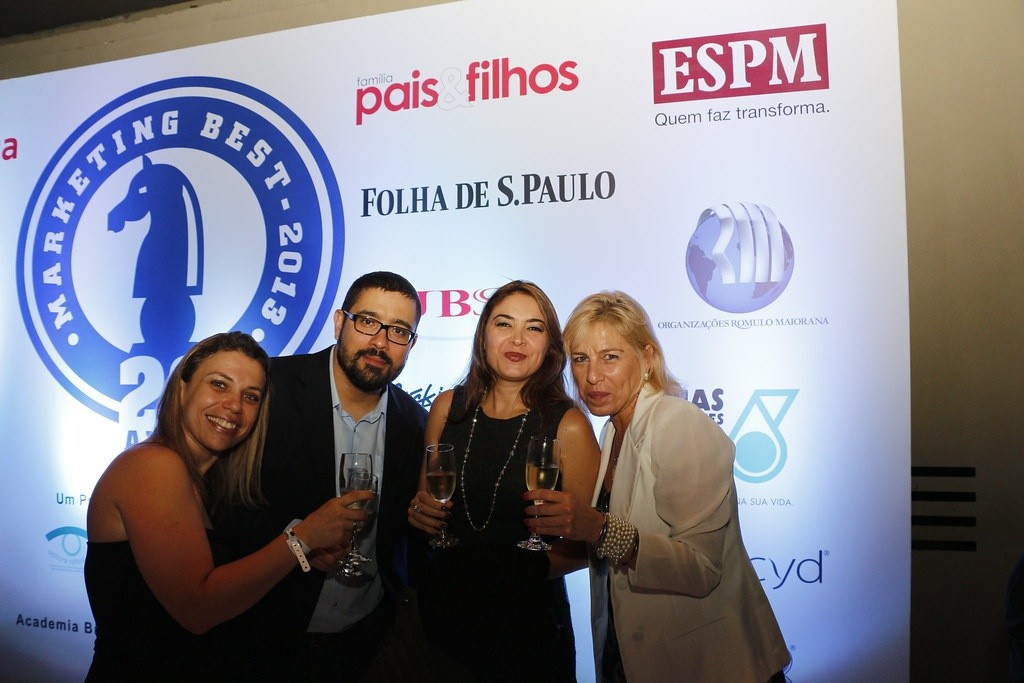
413, 505, 418, 511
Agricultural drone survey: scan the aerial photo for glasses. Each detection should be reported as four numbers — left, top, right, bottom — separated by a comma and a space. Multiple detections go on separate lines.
343, 310, 415, 346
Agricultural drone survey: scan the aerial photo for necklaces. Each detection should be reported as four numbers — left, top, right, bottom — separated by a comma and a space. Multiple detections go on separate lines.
609, 457, 618, 461
460, 387, 530, 533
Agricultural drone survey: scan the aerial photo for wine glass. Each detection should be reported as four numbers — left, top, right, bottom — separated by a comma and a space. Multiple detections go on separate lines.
332, 451, 372, 578
340, 473, 377, 562
518, 436, 561, 551
425, 444, 457, 547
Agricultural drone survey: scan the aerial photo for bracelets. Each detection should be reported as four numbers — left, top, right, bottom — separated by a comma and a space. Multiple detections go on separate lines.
597, 513, 639, 565
285, 528, 311, 573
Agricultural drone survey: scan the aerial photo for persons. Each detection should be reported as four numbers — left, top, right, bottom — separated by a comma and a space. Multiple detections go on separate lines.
84, 330, 377, 683
244, 272, 428, 683
522, 291, 790, 683
406, 281, 601, 683
